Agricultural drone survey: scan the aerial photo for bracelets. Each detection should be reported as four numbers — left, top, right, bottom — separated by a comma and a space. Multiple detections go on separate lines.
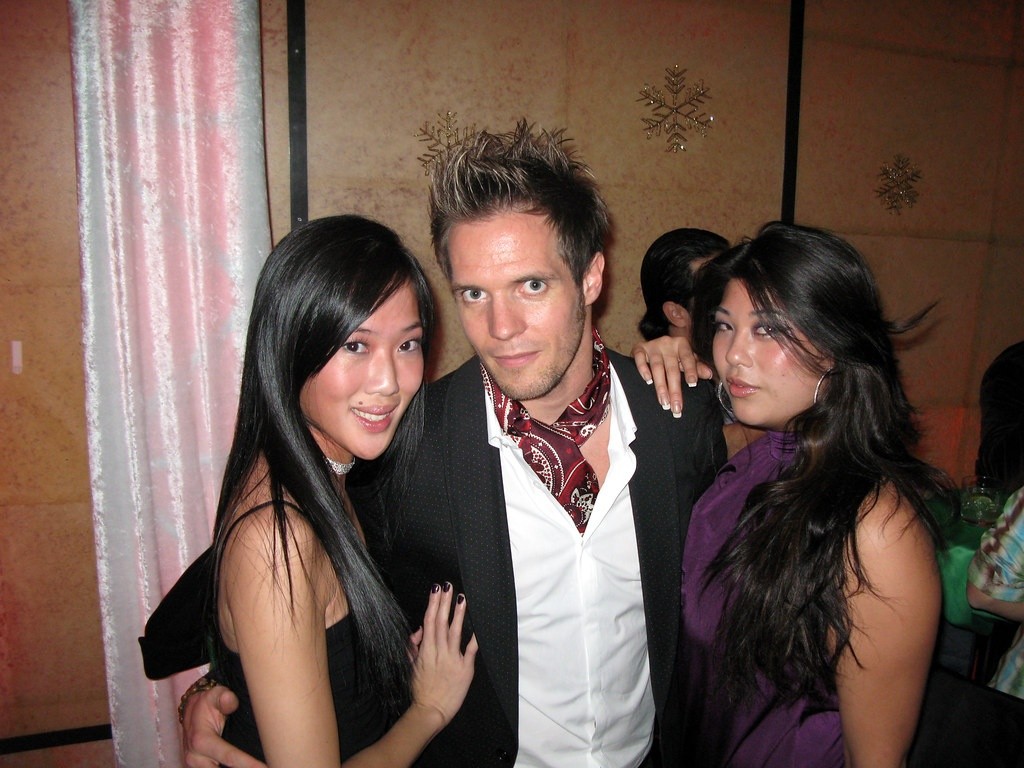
177, 677, 221, 725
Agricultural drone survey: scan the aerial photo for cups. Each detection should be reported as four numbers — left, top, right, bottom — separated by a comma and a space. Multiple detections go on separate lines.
960, 474, 1009, 527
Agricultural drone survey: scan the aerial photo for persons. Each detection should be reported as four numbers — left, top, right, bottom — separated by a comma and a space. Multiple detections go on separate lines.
967, 486, 1024, 699
636, 227, 739, 427
180, 146, 771, 768
210, 215, 478, 768
636, 220, 942, 768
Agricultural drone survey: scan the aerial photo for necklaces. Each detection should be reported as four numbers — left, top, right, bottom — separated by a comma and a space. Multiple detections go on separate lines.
326, 457, 355, 475
